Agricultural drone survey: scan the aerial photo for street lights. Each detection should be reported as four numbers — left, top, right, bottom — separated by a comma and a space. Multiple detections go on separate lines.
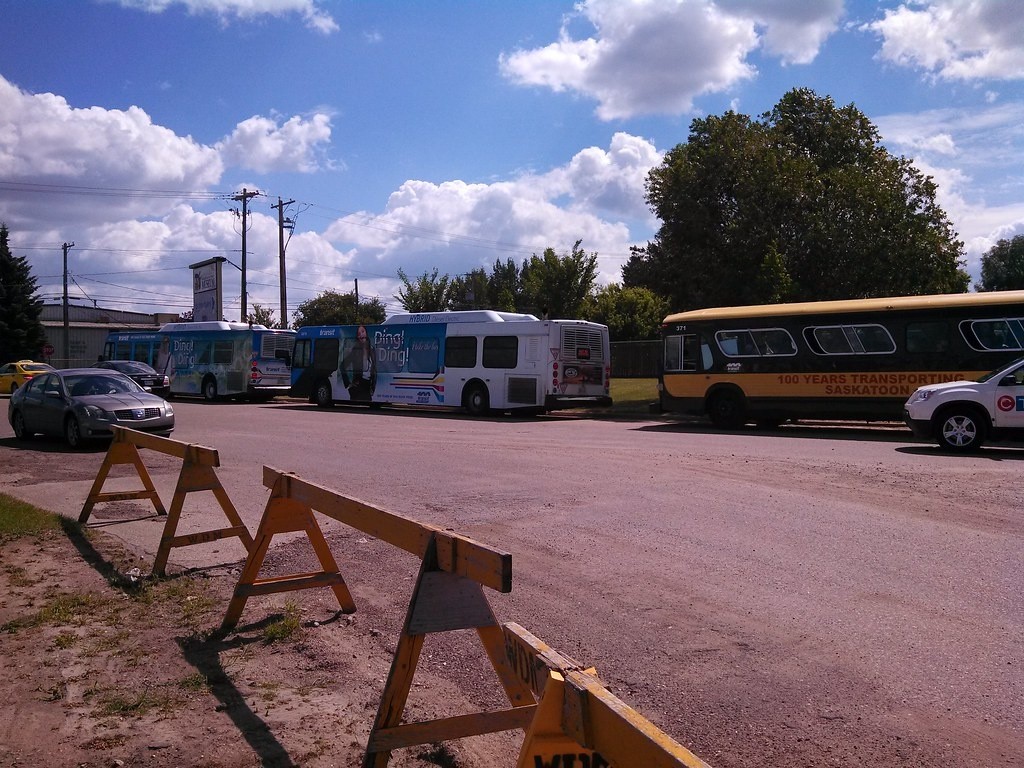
221, 257, 247, 323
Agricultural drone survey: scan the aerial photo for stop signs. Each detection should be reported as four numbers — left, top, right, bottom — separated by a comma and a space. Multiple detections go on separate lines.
43, 345, 55, 355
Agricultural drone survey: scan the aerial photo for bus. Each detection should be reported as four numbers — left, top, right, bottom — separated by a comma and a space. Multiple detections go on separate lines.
609, 339, 666, 409
98, 320, 299, 403
285, 310, 613, 420
661, 289, 1024, 430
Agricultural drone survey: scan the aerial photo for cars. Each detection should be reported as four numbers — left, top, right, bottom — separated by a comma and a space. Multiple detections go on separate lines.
80, 361, 171, 398
0, 359, 60, 395
8, 366, 175, 447
904, 357, 1024, 451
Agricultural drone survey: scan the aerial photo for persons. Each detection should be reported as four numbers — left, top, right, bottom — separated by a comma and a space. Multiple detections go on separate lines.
341, 325, 377, 401
157, 337, 175, 378
